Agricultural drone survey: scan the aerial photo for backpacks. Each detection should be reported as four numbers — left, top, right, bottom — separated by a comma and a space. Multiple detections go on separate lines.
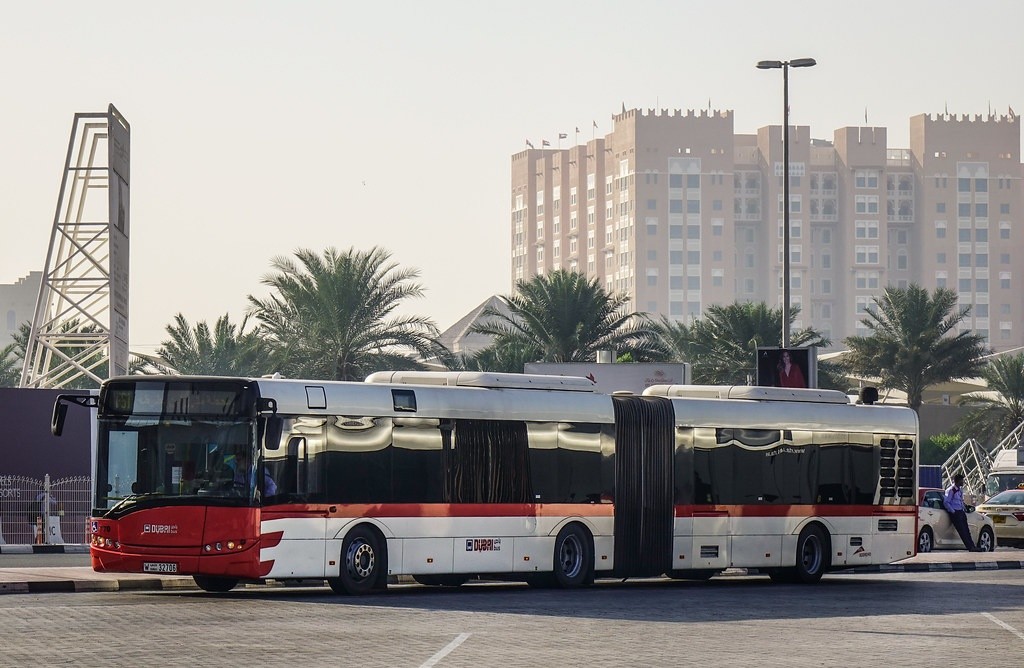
28, 494, 45, 525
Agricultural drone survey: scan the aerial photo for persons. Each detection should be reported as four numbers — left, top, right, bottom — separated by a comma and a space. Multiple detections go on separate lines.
203, 452, 235, 490
944, 474, 980, 552
235, 452, 277, 498
775, 351, 807, 388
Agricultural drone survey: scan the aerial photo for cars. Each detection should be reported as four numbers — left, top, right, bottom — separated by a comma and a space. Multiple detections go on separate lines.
919, 487, 998, 553
974, 489, 1024, 550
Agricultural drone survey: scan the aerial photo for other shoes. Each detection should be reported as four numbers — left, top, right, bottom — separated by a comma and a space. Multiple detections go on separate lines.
969, 547, 982, 552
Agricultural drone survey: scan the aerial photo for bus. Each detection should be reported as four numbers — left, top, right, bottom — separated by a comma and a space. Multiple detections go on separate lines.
48, 350, 918, 595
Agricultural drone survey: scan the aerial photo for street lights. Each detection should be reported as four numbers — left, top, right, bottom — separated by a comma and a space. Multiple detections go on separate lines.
756, 58, 817, 388
